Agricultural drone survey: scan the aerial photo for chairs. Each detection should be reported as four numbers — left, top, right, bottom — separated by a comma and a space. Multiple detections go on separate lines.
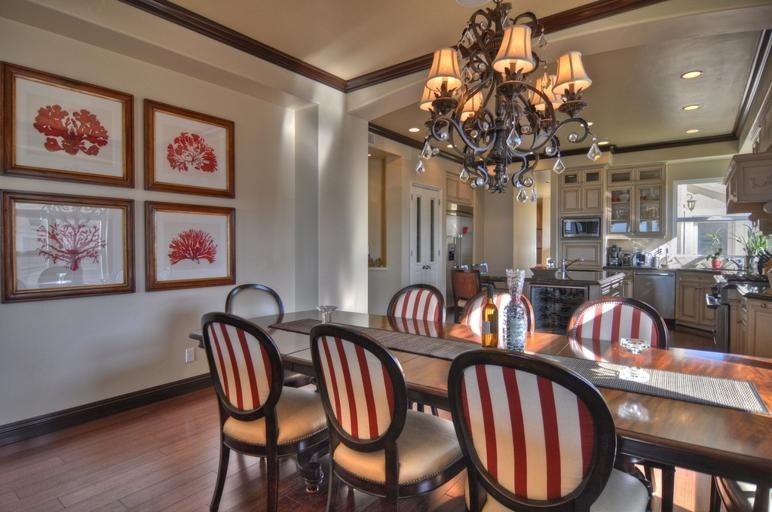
387, 284, 447, 418
225, 284, 318, 389
448, 347, 653, 512
309, 323, 480, 511
461, 290, 536, 333
201, 311, 353, 511
569, 296, 669, 492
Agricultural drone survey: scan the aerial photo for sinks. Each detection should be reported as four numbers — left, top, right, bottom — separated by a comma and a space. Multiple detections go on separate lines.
661, 263, 680, 269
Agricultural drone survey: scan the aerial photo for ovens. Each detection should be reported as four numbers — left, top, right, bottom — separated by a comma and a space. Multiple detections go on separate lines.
706, 293, 729, 354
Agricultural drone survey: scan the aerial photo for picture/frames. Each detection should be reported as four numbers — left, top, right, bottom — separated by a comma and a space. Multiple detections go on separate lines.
143, 201, 236, 292
0, 61, 135, 188
143, 97, 236, 198
0, 188, 136, 304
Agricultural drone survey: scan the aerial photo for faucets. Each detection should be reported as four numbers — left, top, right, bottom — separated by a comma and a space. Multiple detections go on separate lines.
557, 256, 584, 271
666, 248, 669, 264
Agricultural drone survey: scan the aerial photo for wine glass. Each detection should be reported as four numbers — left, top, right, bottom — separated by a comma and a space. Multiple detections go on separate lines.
614, 391, 651, 421
317, 305, 338, 326
617, 338, 654, 381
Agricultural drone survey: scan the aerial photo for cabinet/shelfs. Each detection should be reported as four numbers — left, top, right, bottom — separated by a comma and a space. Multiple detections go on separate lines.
559, 163, 666, 280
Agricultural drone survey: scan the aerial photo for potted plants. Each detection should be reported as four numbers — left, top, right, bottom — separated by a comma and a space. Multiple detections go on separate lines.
705, 229, 723, 269
729, 224, 767, 272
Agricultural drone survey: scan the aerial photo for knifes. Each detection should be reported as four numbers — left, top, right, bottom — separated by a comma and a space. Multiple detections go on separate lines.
755, 248, 772, 264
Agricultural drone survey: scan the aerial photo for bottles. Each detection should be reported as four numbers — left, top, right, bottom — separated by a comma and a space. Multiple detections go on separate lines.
607, 245, 622, 267
623, 253, 633, 267
632, 251, 643, 268
610, 187, 661, 233
481, 267, 527, 356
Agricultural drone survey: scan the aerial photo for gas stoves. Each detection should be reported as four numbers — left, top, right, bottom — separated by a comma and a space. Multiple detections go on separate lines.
710, 273, 769, 297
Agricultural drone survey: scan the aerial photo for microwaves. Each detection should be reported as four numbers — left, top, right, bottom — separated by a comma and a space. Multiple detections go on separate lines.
560, 216, 601, 239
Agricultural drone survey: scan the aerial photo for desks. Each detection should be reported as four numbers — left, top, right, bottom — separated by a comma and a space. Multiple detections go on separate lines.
190, 318, 772, 512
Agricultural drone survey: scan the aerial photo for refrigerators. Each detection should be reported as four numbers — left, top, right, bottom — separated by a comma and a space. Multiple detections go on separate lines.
444, 202, 475, 309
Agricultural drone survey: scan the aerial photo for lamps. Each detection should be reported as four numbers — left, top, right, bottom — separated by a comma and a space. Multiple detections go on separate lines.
416, 0, 603, 205
679, 192, 696, 214
